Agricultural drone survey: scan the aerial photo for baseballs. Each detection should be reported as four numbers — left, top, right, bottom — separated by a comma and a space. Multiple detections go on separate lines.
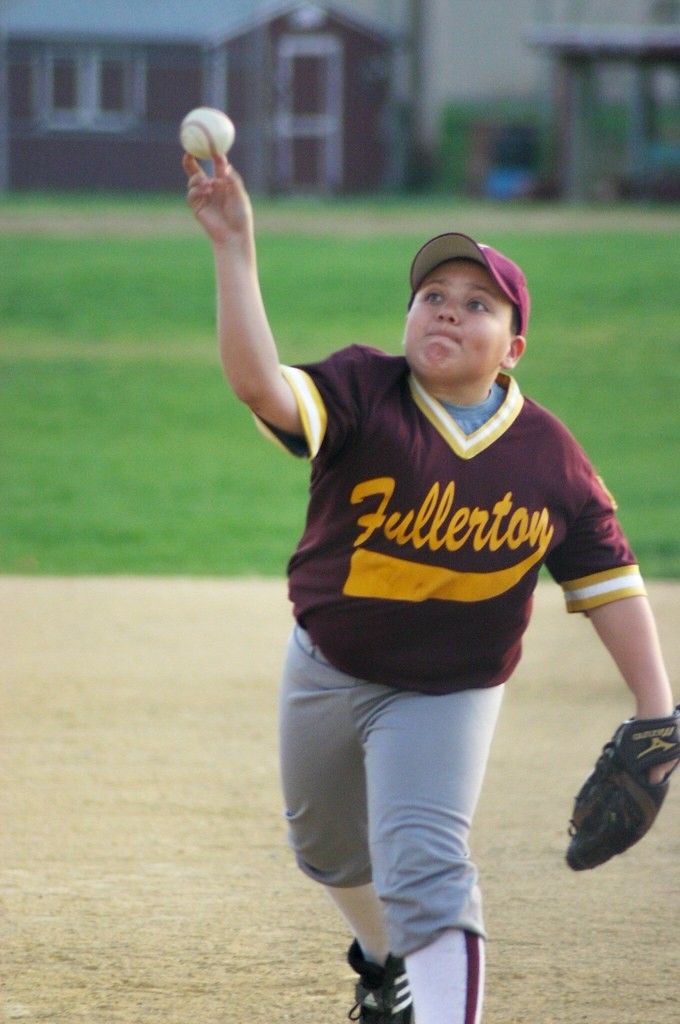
178, 105, 237, 163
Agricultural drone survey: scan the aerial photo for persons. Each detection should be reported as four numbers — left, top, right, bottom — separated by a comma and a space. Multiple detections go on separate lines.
184, 149, 680, 1023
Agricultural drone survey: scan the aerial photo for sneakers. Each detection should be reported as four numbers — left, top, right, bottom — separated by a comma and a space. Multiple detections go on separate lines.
348, 937, 413, 1024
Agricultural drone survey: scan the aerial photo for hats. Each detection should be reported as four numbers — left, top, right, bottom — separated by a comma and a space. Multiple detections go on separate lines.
410, 232, 531, 336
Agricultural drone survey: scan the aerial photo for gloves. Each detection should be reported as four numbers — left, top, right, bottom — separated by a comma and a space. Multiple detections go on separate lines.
565, 705, 680, 871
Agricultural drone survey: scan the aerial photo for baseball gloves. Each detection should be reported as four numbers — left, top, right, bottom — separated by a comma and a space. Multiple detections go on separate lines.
563, 710, 679, 874
